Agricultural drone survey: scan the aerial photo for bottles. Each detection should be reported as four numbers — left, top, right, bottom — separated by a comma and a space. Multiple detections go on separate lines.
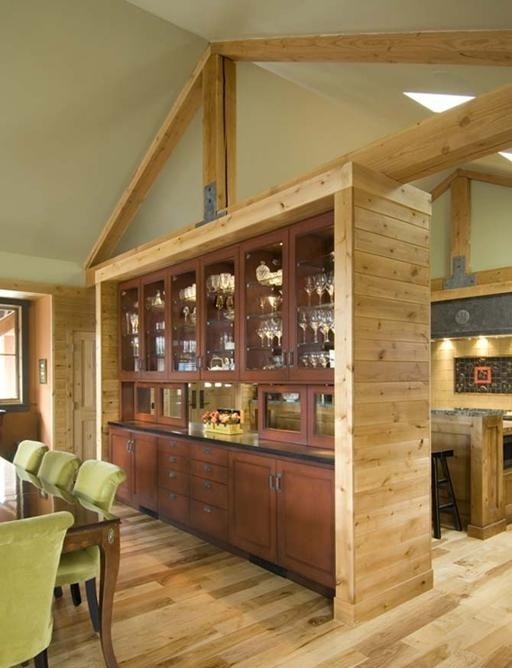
255, 262, 270, 281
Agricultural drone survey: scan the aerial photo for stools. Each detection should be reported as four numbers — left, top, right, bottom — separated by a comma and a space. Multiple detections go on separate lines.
432, 448, 462, 540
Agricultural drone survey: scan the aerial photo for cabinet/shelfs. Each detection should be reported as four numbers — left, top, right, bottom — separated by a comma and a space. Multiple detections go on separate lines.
116, 208, 334, 452
227, 448, 334, 591
158, 436, 190, 526
189, 441, 228, 540
108, 428, 158, 514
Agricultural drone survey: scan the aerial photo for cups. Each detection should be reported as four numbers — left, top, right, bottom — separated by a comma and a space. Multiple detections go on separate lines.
179, 283, 197, 300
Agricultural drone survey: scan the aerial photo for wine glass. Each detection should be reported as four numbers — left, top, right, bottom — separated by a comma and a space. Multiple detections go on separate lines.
297, 271, 334, 367
255, 294, 282, 346
130, 313, 138, 334
207, 273, 235, 292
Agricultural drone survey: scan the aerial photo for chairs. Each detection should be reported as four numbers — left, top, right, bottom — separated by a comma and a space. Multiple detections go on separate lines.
0, 439, 128, 668
